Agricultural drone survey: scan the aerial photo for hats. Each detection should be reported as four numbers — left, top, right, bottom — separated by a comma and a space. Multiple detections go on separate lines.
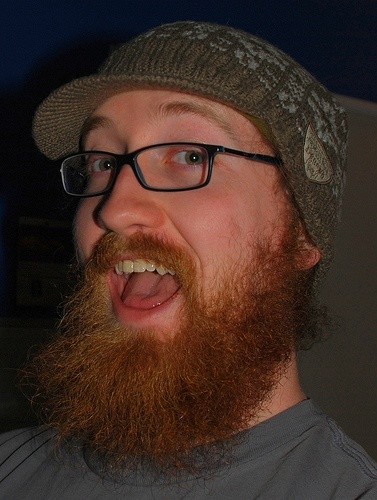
34, 19, 347, 257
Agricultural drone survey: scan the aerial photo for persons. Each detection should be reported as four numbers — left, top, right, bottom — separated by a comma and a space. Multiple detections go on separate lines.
0, 21, 377, 499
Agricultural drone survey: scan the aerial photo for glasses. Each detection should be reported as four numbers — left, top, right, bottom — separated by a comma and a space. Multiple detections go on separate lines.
56, 142, 281, 198
